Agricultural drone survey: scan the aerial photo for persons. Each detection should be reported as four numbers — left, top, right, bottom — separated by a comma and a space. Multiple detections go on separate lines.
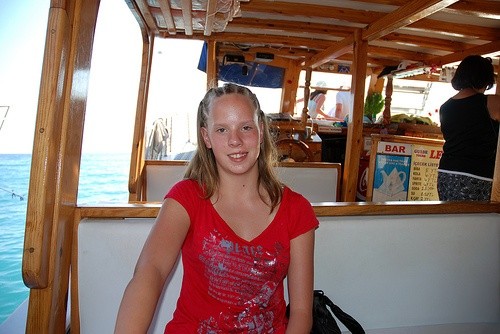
437, 54, 500, 202
335, 85, 351, 119
114, 83, 319, 334
294, 89, 333, 119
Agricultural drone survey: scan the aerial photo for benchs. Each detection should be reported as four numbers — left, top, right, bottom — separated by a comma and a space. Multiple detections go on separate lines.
72, 199, 500, 334
143, 159, 343, 201
